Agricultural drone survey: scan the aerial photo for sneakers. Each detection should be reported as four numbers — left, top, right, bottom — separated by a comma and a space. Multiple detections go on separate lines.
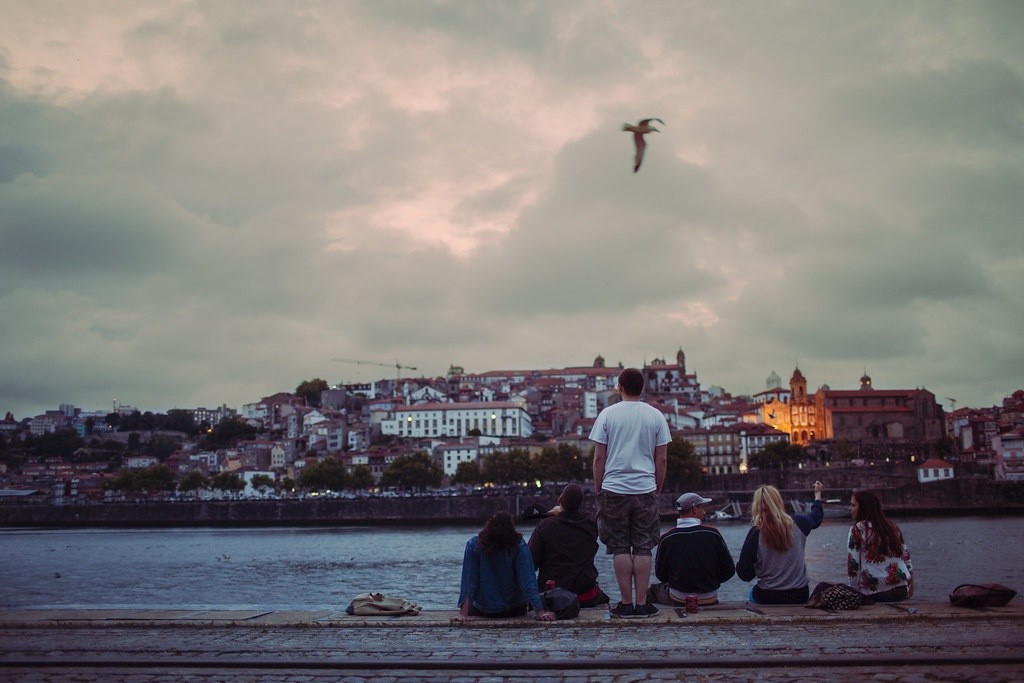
633, 602, 659, 618
609, 601, 634, 619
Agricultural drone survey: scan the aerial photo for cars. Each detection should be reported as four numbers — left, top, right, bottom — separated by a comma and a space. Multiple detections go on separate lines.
167, 485, 507, 504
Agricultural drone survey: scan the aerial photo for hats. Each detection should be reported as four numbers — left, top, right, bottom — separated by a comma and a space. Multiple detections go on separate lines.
676, 492, 713, 511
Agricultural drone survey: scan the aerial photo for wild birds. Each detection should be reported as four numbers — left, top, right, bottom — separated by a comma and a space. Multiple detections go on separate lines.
621, 118, 665, 174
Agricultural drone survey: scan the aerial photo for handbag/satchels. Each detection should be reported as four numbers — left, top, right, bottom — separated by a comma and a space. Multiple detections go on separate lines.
538, 588, 581, 620
948, 582, 1018, 608
803, 580, 876, 611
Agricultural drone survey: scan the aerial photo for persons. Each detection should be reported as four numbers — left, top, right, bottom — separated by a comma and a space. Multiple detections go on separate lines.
646, 493, 737, 606
846, 489, 916, 601
526, 481, 612, 610
450, 511, 558, 624
734, 480, 824, 605
588, 369, 673, 619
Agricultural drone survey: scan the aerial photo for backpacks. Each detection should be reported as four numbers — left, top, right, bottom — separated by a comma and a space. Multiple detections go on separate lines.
346, 592, 423, 616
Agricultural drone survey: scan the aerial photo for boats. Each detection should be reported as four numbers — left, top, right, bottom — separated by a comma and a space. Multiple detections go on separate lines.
789, 498, 853, 519
704, 497, 744, 520
